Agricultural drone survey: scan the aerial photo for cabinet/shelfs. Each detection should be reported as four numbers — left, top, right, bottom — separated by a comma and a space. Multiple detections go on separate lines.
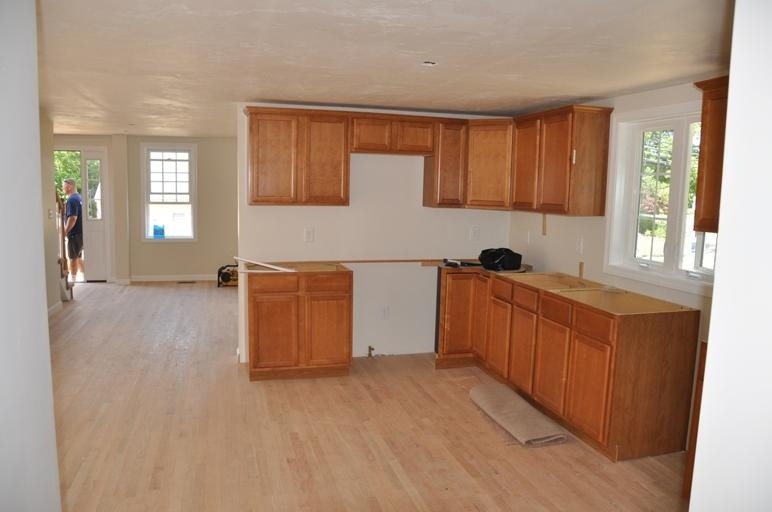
485, 273, 612, 403
433, 265, 532, 370
511, 106, 614, 218
245, 272, 354, 381
348, 112, 434, 156
532, 290, 702, 463
422, 118, 514, 210
243, 106, 350, 207
692, 73, 730, 233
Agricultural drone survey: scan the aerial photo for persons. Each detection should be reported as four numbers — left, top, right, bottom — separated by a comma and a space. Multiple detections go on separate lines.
62, 177, 85, 283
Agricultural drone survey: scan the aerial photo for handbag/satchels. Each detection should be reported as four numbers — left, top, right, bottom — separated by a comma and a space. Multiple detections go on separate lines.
478, 246, 522, 271
